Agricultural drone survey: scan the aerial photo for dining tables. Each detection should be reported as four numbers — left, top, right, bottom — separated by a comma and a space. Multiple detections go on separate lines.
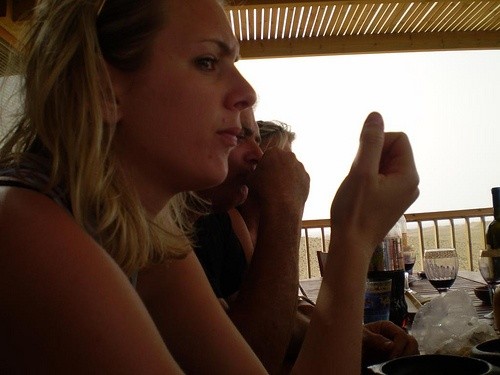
298, 269, 500, 375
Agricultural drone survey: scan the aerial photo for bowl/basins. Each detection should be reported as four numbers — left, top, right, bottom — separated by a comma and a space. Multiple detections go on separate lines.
474, 286, 493, 304
472, 338, 500, 366
367, 353, 494, 375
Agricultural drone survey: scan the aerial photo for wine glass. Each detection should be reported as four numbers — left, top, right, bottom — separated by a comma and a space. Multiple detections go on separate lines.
478, 249, 499, 318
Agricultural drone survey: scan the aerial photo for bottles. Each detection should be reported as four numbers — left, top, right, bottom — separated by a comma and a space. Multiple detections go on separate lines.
484, 187, 500, 278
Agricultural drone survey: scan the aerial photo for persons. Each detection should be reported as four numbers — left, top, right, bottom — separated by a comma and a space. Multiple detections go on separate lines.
0, 0, 419, 375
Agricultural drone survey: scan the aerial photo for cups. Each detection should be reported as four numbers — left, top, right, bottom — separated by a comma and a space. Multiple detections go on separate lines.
424, 249, 459, 293
364, 278, 392, 323
404, 252, 416, 273
